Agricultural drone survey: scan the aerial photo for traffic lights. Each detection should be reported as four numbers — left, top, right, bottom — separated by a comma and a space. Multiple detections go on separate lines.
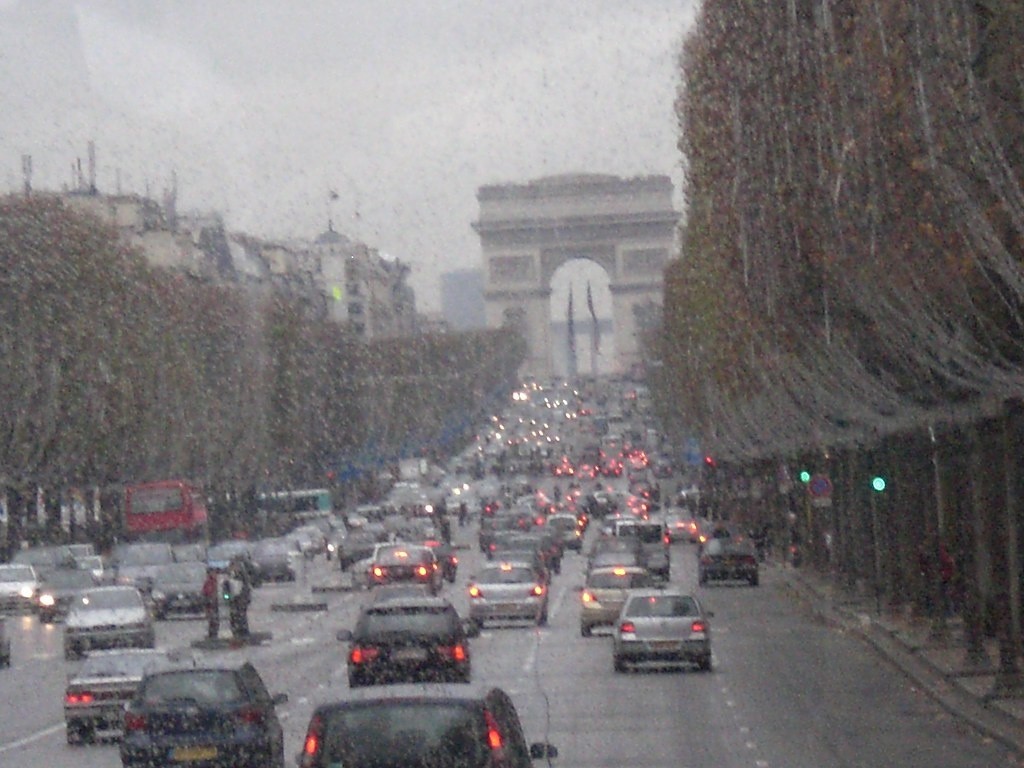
799, 469, 815, 486
869, 476, 896, 495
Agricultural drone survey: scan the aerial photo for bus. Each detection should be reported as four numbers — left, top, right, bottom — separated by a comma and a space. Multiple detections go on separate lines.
122, 477, 207, 539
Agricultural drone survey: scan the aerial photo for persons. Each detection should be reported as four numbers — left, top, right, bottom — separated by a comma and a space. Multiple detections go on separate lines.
202, 568, 250, 637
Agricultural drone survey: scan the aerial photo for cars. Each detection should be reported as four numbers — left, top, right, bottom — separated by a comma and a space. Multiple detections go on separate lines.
295, 682, 559, 768
466, 477, 589, 629
63, 585, 155, 659
599, 425, 676, 486
588, 485, 768, 584
335, 486, 481, 596
335, 597, 480, 687
576, 565, 718, 673
63, 647, 290, 768
0, 524, 329, 615
